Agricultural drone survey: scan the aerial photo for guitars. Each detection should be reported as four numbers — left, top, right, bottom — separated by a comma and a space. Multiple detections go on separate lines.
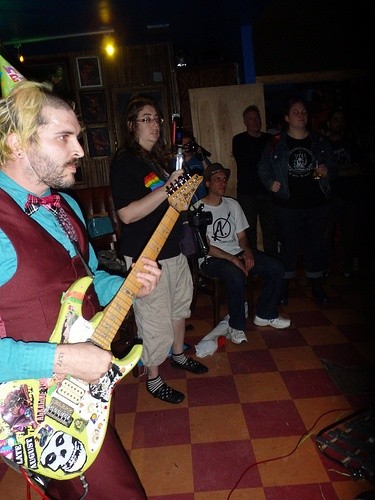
0, 175, 205, 480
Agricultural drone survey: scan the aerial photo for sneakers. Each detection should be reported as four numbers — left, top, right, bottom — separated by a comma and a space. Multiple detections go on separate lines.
227, 327, 248, 344
253, 315, 291, 329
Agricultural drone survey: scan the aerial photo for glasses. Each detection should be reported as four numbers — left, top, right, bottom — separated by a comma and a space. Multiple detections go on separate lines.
137, 116, 160, 124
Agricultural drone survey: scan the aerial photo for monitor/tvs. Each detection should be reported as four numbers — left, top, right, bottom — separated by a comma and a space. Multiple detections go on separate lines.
85, 215, 114, 239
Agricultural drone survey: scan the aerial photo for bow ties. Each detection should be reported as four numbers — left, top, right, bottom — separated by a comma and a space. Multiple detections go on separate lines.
24, 192, 59, 215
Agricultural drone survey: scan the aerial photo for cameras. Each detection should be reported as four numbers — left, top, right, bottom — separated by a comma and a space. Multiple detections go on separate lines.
185, 142, 198, 153
187, 208, 213, 227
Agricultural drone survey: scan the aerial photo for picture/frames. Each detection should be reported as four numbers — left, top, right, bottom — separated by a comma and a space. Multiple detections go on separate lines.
86, 126, 112, 159
79, 91, 109, 124
112, 85, 172, 156
77, 56, 103, 89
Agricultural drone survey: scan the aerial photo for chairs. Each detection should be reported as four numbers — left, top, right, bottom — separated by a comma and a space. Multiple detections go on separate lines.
190, 247, 256, 326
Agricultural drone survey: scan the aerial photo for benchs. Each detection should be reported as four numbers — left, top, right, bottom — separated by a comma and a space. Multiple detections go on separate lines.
74, 186, 119, 247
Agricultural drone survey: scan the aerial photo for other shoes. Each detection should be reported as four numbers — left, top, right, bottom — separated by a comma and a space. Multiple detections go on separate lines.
146, 376, 184, 403
170, 356, 208, 374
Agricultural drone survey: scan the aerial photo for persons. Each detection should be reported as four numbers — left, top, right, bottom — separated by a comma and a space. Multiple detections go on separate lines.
47, 64, 68, 99
257, 96, 338, 306
188, 162, 291, 345
319, 102, 366, 279
82, 64, 99, 86
84, 93, 106, 122
110, 98, 208, 404
90, 125, 108, 156
169, 134, 211, 205
233, 104, 274, 255
0, 82, 162, 500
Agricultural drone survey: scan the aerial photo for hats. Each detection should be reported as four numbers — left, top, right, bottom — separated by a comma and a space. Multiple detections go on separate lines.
203, 162, 230, 180
